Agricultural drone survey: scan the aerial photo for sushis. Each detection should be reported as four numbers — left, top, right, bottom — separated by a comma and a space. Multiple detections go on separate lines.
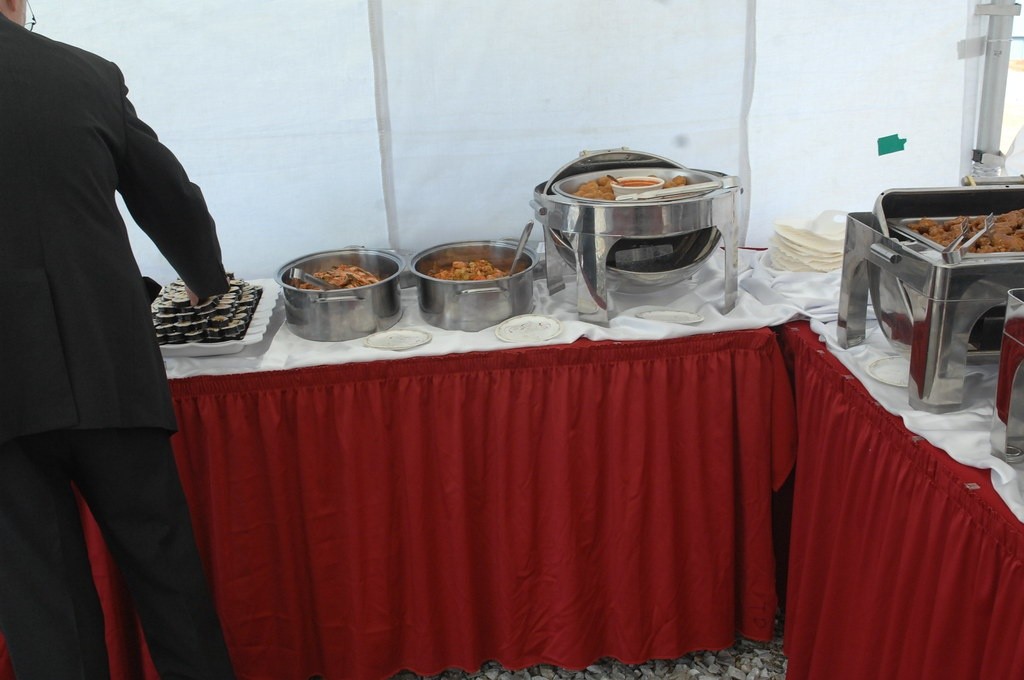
149, 272, 260, 343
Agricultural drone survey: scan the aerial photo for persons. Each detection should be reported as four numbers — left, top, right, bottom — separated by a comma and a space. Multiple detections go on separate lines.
0, 1, 235, 680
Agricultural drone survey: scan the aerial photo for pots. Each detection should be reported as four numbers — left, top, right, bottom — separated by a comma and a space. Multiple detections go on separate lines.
408, 240, 543, 332
276, 244, 407, 342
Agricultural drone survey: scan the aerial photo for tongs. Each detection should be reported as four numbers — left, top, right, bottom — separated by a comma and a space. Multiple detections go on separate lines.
941, 212, 995, 264
289, 268, 338, 292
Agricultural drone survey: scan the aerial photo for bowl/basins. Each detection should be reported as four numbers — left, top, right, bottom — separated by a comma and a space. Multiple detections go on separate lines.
610, 176, 665, 199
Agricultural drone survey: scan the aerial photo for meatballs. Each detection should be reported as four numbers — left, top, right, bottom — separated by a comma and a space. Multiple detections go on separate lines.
572, 175, 615, 200
663, 175, 686, 188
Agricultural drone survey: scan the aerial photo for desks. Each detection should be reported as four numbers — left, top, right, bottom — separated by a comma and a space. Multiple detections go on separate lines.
0, 248, 1024, 680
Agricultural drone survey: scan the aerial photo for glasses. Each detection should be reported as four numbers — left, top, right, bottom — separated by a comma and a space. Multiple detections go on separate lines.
24, 0, 36, 32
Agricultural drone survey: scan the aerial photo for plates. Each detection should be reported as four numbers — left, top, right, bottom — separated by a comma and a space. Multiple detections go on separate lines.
364, 329, 433, 351
866, 355, 911, 388
495, 313, 564, 343
635, 310, 705, 324
